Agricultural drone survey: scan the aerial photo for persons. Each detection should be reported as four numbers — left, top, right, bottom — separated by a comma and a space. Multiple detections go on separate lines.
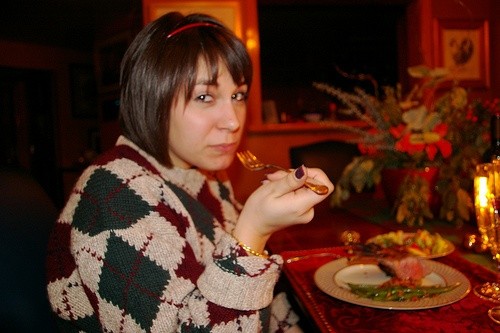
47, 12, 334, 333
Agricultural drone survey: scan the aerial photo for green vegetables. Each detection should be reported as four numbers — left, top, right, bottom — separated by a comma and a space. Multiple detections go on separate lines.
345, 281, 460, 302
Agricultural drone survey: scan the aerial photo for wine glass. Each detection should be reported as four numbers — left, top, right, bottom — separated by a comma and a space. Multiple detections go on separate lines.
472, 164, 500, 303
487, 156, 499, 327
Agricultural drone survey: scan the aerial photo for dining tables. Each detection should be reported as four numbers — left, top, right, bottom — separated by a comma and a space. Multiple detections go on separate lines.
274, 236, 500, 333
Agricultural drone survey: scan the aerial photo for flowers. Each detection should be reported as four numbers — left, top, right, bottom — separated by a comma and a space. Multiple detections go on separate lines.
303, 62, 500, 229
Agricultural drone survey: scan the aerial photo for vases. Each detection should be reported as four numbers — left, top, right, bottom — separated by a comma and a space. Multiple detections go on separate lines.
380, 160, 439, 219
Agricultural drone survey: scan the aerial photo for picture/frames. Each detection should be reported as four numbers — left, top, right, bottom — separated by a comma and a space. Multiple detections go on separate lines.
431, 14, 492, 88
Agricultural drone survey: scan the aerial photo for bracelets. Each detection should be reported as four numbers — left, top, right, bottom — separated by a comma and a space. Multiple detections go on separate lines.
230, 228, 268, 259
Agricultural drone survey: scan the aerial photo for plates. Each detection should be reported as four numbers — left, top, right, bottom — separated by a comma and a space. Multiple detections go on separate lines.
369, 231, 454, 258
313, 254, 470, 309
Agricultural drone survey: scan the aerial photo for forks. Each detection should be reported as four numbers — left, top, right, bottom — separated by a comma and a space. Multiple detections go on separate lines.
236, 150, 329, 195
